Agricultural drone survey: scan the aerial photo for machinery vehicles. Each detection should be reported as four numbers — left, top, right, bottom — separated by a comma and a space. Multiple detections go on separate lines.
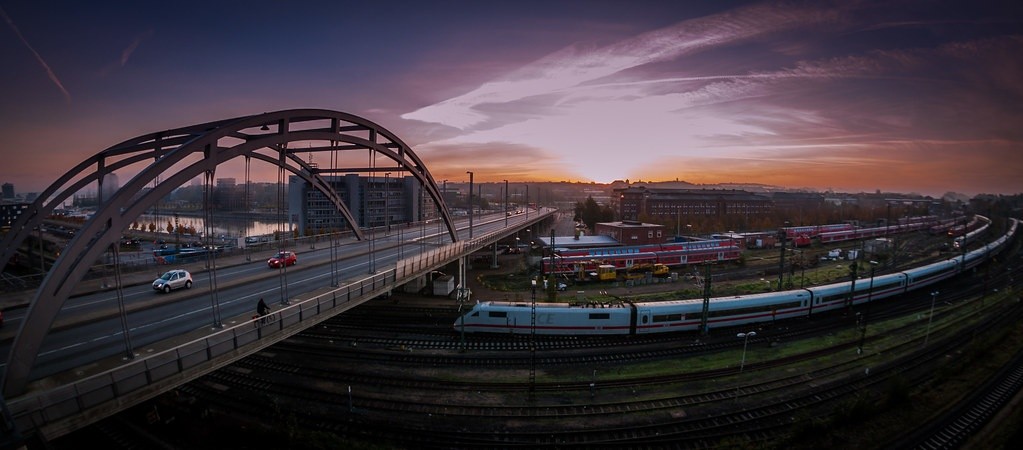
579, 262, 617, 283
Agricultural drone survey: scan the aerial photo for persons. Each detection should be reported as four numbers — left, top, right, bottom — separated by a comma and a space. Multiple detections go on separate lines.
257, 298, 271, 324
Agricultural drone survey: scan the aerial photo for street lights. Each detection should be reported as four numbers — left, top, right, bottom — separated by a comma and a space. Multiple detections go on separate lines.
737, 332, 756, 371
385, 172, 391, 234
503, 180, 508, 226
861, 260, 878, 349
525, 185, 528, 218
687, 225, 692, 245
467, 171, 472, 239
729, 231, 734, 260
444, 180, 448, 197
924, 292, 939, 345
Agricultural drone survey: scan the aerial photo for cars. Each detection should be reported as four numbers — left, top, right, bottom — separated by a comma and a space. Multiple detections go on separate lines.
152, 269, 192, 293
268, 251, 298, 268
544, 283, 567, 291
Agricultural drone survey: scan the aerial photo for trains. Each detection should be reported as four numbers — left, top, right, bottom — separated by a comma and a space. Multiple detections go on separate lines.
540, 239, 742, 275
453, 217, 1019, 335
780, 209, 992, 250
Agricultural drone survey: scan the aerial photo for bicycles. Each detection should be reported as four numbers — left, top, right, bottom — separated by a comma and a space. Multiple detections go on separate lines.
252, 308, 275, 328
